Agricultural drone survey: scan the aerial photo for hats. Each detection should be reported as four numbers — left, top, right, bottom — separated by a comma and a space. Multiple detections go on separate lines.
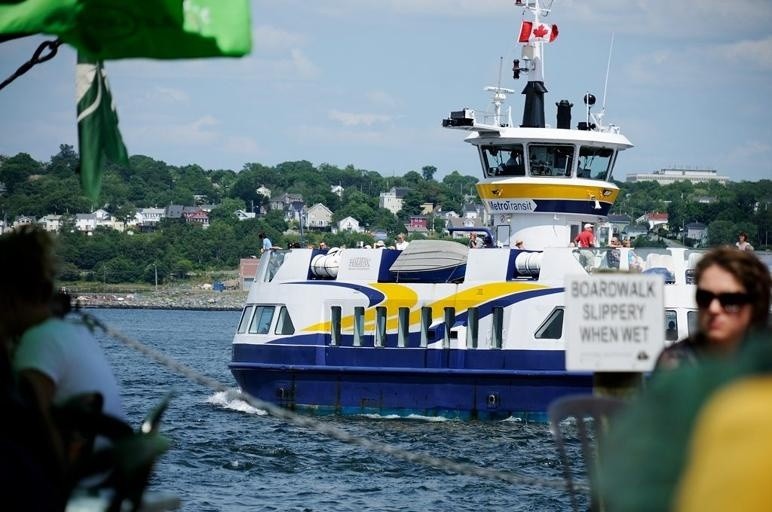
585, 223, 594, 229
364, 239, 396, 250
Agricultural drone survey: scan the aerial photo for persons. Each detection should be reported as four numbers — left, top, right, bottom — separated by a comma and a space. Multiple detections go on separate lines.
666, 319, 677, 341
506, 151, 519, 167
0, 222, 129, 512
651, 247, 770, 380
735, 231, 752, 252
577, 159, 583, 177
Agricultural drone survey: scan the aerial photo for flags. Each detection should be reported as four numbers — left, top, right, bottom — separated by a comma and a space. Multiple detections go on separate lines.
518, 20, 560, 45
76, 61, 133, 207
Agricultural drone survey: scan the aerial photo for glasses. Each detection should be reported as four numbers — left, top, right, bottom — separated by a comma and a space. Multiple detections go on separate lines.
696, 286, 758, 312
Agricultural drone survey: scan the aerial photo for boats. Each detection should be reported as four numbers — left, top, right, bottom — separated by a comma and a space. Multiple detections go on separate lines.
227, 0, 720, 423
